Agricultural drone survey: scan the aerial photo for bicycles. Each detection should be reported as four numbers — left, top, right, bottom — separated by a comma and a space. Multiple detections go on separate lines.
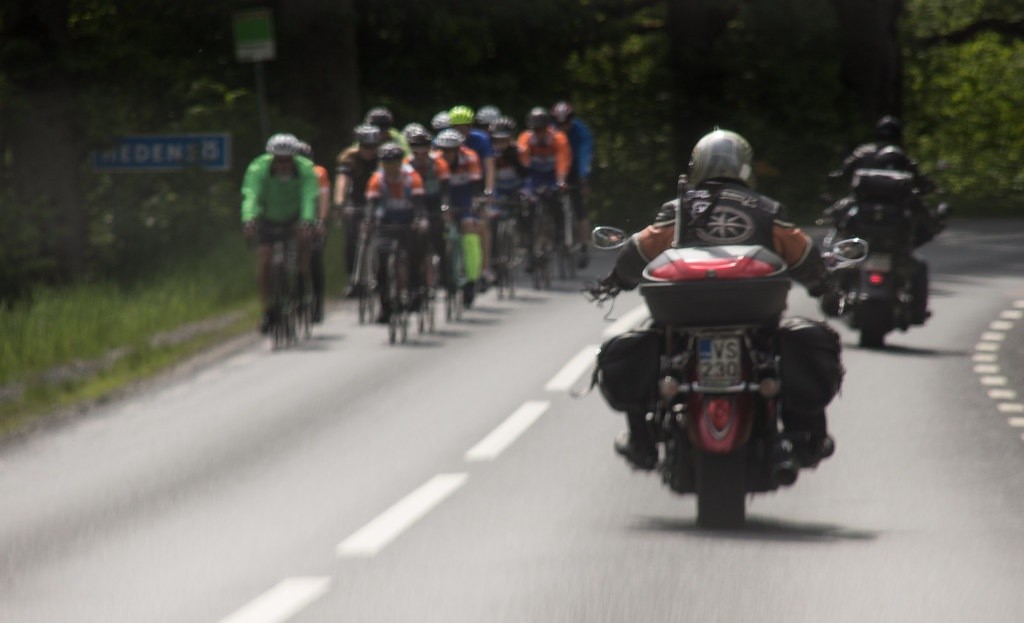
250, 186, 592, 352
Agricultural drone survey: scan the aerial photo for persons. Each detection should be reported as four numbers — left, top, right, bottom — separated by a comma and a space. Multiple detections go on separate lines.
598, 130, 844, 472
825, 117, 947, 327
225, 99, 598, 334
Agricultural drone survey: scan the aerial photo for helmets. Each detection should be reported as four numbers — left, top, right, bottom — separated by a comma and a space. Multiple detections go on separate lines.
526, 106, 549, 129
266, 132, 314, 161
687, 130, 753, 186
353, 106, 516, 161
551, 101, 572, 122
874, 146, 907, 171
874, 115, 902, 142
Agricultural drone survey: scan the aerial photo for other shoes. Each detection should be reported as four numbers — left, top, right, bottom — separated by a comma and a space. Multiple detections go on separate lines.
615, 429, 659, 468
792, 434, 834, 468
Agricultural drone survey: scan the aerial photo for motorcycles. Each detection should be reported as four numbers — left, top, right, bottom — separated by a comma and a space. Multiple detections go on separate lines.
593, 226, 868, 530
813, 186, 951, 348
